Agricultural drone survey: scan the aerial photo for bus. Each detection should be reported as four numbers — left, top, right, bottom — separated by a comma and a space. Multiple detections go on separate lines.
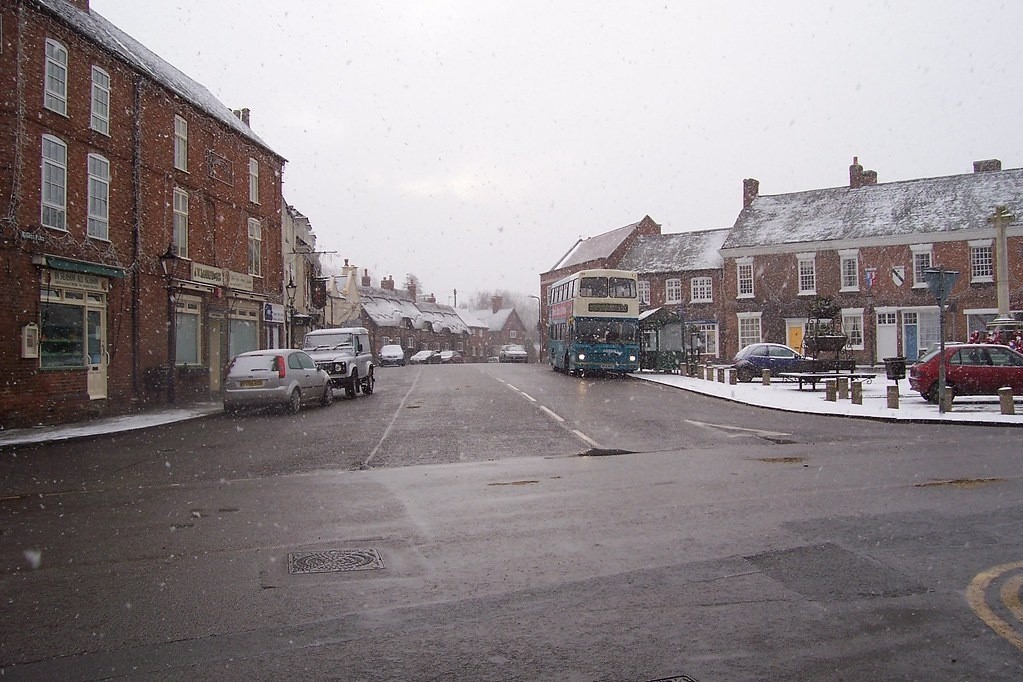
548, 268, 640, 378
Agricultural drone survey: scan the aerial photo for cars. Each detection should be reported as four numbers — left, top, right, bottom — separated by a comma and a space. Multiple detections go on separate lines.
410, 350, 442, 364
440, 350, 464, 363
732, 343, 818, 383
222, 348, 335, 412
908, 343, 1023, 403
379, 345, 407, 366
499, 345, 528, 363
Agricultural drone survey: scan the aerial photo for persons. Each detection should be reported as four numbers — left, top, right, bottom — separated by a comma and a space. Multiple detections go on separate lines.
970, 341, 993, 365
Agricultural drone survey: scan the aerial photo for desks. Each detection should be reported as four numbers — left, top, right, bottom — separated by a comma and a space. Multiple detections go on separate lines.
779, 372, 877, 391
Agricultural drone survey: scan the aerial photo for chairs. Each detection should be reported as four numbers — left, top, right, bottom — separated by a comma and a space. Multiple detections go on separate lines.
968, 350, 990, 364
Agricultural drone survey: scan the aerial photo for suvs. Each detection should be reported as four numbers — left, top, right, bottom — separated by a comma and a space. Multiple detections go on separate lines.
300, 327, 375, 398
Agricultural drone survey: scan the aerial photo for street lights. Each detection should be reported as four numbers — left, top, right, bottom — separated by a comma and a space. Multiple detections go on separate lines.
285, 275, 298, 350
160, 241, 181, 411
527, 294, 542, 362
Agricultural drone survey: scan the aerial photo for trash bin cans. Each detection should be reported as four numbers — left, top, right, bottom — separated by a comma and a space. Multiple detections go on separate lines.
883, 357, 906, 380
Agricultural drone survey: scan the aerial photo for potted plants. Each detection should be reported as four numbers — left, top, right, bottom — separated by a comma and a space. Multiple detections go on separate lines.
804, 323, 848, 352
810, 295, 842, 317
